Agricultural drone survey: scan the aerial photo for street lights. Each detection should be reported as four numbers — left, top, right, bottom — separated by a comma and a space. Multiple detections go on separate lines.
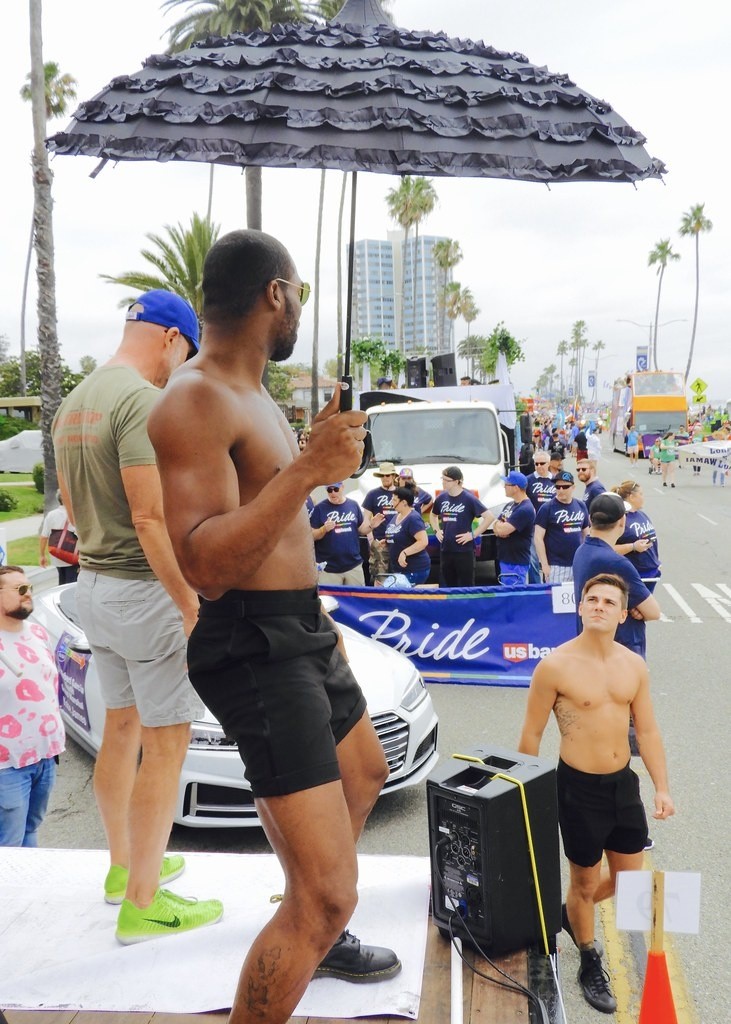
612, 318, 688, 371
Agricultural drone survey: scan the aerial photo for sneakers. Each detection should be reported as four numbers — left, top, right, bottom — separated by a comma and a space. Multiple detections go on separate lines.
561, 903, 604, 957
114, 888, 224, 945
576, 963, 616, 1012
103, 853, 185, 904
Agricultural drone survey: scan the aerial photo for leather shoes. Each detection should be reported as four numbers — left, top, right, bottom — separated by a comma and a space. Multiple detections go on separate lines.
310, 927, 403, 983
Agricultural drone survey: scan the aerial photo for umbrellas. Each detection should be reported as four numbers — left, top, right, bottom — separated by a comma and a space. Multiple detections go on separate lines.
43, 0, 668, 482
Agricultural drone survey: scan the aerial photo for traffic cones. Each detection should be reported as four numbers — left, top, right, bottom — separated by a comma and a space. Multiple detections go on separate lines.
639, 950, 682, 1024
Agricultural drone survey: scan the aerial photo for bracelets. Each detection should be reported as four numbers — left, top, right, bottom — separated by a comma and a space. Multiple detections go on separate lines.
369, 524, 373, 529
633, 542, 636, 552
472, 532, 474, 539
434, 528, 439, 534
402, 550, 407, 555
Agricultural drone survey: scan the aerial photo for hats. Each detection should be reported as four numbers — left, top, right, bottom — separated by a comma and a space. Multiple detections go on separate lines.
550, 471, 574, 484
373, 462, 399, 478
501, 471, 527, 489
125, 289, 200, 359
400, 468, 413, 477
590, 490, 632, 523
551, 451, 565, 460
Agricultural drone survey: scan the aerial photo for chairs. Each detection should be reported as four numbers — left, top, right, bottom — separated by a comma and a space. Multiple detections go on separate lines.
452, 415, 493, 458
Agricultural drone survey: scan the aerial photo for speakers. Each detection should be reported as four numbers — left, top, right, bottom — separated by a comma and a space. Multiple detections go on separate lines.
430, 352, 457, 388
425, 745, 564, 960
407, 356, 425, 388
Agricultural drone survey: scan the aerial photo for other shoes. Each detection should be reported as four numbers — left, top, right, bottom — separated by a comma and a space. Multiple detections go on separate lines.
671, 483, 675, 488
643, 839, 655, 849
663, 483, 667, 486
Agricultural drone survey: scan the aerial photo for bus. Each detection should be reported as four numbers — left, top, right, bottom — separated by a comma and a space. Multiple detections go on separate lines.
609, 369, 693, 459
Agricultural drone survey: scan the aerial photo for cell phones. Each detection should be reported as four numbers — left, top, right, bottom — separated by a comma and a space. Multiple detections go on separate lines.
646, 537, 656, 544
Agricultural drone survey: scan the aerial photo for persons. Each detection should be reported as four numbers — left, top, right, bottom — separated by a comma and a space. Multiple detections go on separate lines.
147, 228, 400, 1024
52, 290, 220, 947
460, 377, 470, 385
624, 409, 731, 487
516, 574, 676, 1014
309, 481, 386, 588
0, 567, 66, 847
534, 420, 589, 479
292, 428, 309, 451
390, 466, 606, 585
525, 451, 557, 585
39, 488, 81, 584
362, 461, 399, 587
612, 480, 661, 594
377, 378, 393, 389
573, 490, 660, 851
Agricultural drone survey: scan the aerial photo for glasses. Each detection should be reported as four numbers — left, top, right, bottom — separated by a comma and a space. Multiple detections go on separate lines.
0, 584, 33, 595
265, 278, 311, 306
504, 483, 515, 488
535, 462, 546, 466
327, 487, 339, 493
555, 485, 570, 490
440, 477, 453, 483
576, 468, 589, 472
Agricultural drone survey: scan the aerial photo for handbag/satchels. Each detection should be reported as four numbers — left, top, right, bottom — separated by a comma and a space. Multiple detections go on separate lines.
48, 518, 79, 565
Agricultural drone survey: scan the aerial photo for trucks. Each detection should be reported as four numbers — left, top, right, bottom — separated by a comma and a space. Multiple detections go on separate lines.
339, 383, 533, 564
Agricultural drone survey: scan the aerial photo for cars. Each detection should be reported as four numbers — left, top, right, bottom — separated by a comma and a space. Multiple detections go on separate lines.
26, 580, 443, 829
579, 412, 604, 433
